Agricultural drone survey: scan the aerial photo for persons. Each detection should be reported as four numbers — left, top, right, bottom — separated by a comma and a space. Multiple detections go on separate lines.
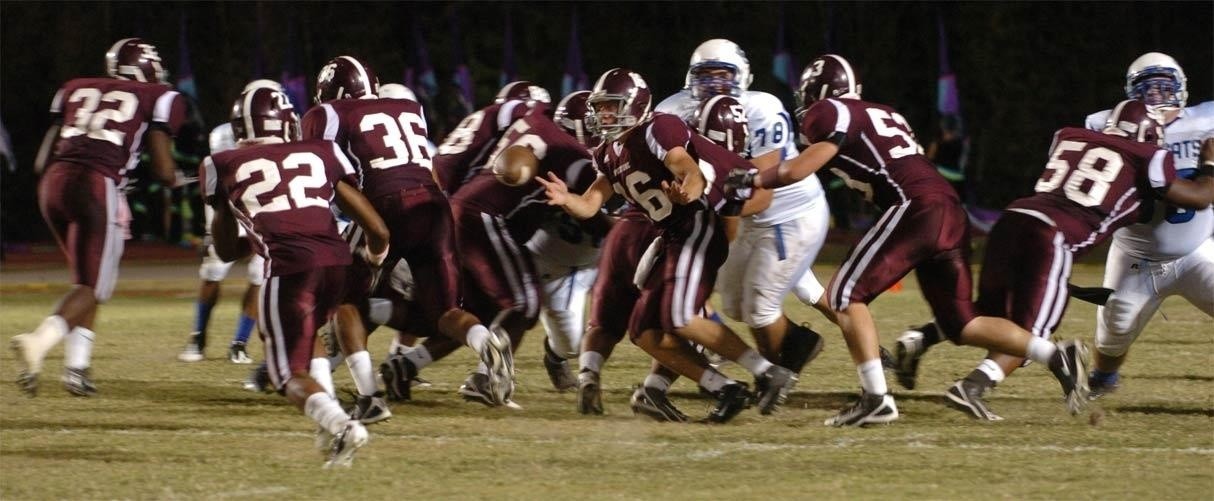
1, 30, 1214, 475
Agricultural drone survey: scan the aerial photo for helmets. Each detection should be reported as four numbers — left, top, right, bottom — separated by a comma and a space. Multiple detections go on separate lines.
1103, 51, 1188, 141
104, 37, 169, 85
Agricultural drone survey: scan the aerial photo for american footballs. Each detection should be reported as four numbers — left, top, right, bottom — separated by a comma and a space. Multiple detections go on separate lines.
492, 145, 539, 187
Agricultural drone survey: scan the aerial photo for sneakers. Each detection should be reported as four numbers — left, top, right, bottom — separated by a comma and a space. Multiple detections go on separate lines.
7, 335, 48, 398
59, 369, 96, 396
